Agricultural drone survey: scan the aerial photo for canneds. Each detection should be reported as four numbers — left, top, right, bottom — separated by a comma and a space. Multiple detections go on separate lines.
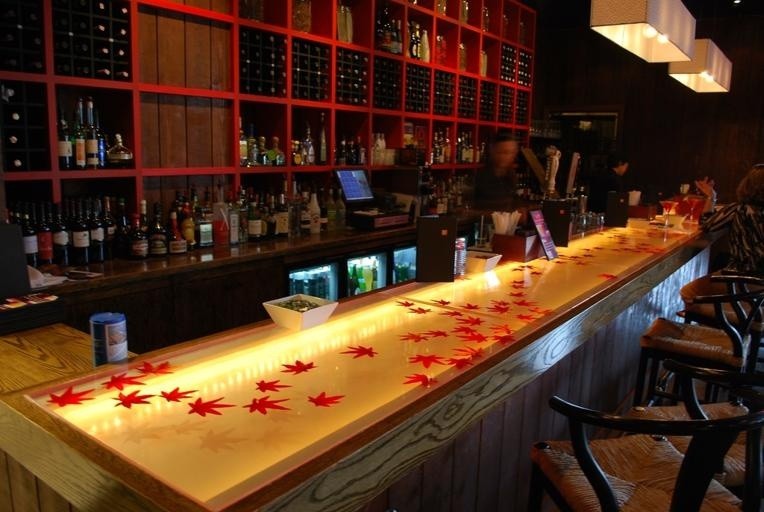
89, 312, 130, 366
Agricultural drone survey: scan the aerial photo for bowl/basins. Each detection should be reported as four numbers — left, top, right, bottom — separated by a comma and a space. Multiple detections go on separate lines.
262, 293, 338, 331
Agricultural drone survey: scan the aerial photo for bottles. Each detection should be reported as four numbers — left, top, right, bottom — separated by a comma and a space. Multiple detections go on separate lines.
568, 187, 585, 213
291, 39, 329, 102
482, 7, 489, 31
462, 1, 469, 21
499, 87, 528, 124
436, 34, 448, 65
459, 43, 466, 72
458, 77, 475, 118
419, 169, 538, 214
433, 70, 455, 115
287, 257, 414, 301
375, 59, 400, 111
336, 5, 354, 43
1, 89, 133, 172
531, 119, 562, 137
240, 29, 286, 95
503, 14, 512, 38
376, 10, 429, 62
336, 50, 367, 105
481, 51, 487, 76
500, 46, 532, 88
438, 1, 446, 15
0, 1, 131, 83
519, 23, 527, 45
405, 64, 429, 113
480, 82, 495, 122
8, 181, 346, 268
235, 110, 488, 167
292, 1, 310, 32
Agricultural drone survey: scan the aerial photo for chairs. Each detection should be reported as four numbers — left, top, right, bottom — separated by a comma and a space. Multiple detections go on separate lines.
526, 379, 764, 511
637, 267, 764, 377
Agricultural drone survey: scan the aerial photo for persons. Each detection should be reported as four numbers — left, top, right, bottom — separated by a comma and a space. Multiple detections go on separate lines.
606, 153, 670, 206
682, 162, 764, 319
472, 137, 541, 209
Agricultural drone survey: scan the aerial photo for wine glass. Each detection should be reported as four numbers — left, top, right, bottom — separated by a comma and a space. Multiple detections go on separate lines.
684, 197, 699, 222
658, 200, 677, 229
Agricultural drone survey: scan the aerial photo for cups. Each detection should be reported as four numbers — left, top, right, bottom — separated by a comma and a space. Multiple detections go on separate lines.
569, 212, 607, 234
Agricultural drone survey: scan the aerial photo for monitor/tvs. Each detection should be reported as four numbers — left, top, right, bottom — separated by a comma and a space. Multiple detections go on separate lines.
334, 168, 374, 203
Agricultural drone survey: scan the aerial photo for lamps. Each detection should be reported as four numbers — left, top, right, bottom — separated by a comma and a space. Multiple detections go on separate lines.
666, 39, 732, 94
589, 0, 697, 65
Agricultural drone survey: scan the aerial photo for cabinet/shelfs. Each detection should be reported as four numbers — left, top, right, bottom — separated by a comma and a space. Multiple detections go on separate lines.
63, 214, 478, 356
0, 0, 537, 263
